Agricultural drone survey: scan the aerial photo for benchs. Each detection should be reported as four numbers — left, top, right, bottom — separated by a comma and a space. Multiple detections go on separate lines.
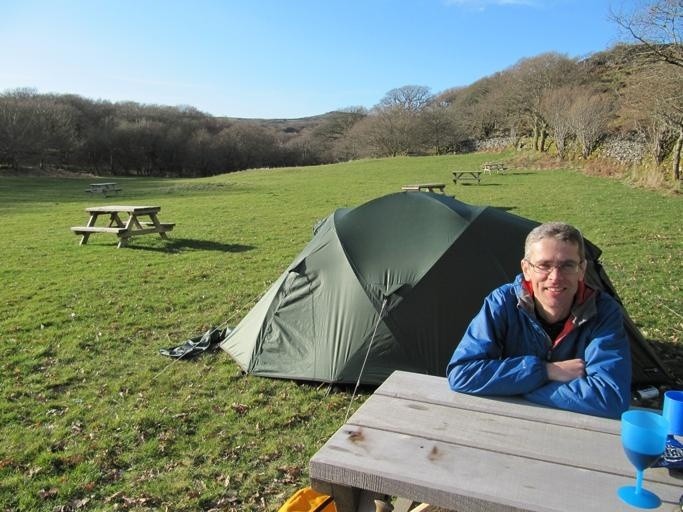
453, 177, 481, 184
120, 221, 176, 239
71, 226, 129, 249
110, 188, 122, 195
85, 188, 107, 198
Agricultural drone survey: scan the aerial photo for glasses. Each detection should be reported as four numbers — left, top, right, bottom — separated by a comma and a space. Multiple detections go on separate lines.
525, 259, 586, 274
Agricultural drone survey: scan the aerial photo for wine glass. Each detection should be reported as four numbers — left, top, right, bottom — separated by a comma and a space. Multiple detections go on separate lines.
618, 410, 669, 509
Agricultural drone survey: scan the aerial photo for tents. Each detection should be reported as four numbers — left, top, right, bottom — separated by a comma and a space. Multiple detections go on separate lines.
216, 190, 673, 391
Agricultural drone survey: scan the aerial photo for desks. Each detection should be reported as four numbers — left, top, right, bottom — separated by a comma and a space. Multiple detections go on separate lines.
79, 206, 167, 247
89, 183, 117, 197
452, 171, 482, 185
308, 371, 683, 512
401, 183, 446, 193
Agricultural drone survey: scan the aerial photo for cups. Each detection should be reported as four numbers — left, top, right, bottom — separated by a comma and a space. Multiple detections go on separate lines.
662, 390, 683, 436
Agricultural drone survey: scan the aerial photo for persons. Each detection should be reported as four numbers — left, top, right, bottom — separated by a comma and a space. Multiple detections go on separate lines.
447, 223, 634, 418
